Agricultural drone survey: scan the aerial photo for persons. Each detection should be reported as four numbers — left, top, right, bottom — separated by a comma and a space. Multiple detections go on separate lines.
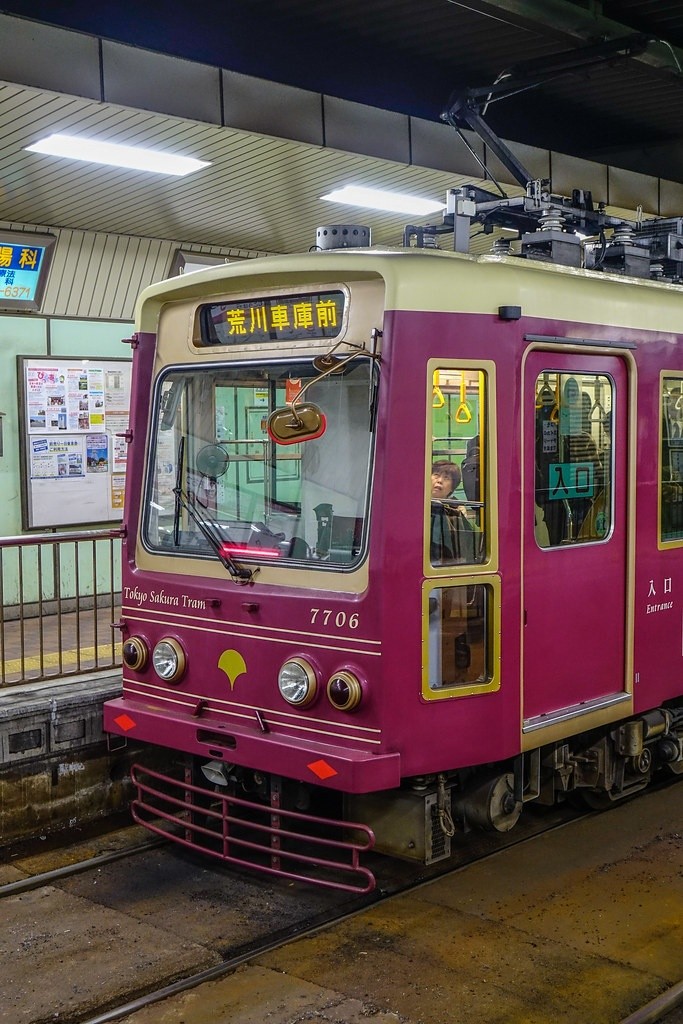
662, 388, 683, 526
466, 433, 481, 458
536, 389, 613, 546
429, 459, 472, 668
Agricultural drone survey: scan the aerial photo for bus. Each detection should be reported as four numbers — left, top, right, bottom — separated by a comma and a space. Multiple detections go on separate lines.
100, 30, 682, 897
